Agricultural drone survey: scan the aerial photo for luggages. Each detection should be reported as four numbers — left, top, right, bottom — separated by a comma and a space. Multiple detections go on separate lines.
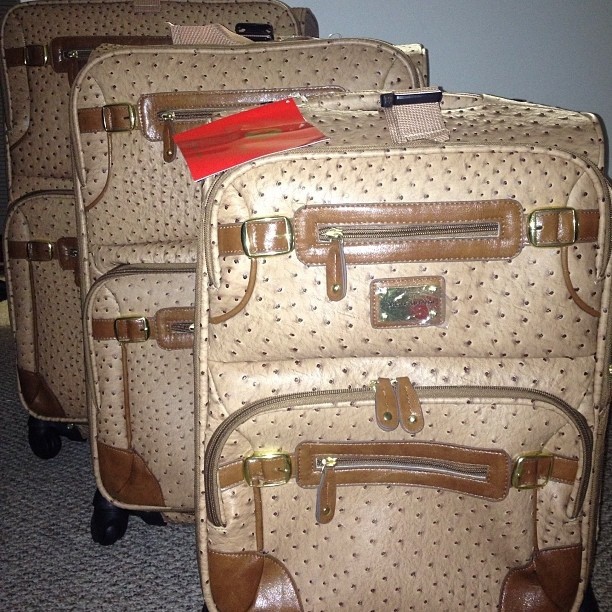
68, 31, 430, 547
0, 0, 320, 461
192, 86, 612, 612
